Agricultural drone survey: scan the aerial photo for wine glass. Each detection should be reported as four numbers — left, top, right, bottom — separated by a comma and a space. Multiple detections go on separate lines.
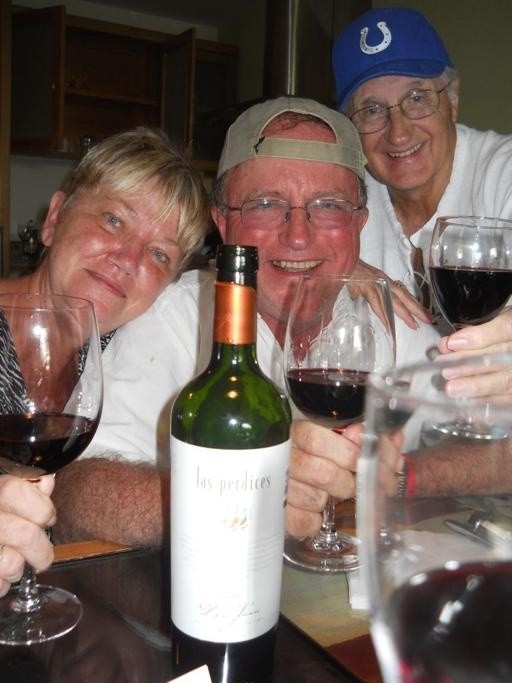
0, 288, 109, 643
283, 261, 399, 571
420, 213, 511, 439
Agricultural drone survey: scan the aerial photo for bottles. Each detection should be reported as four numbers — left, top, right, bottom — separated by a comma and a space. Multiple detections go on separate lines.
165, 242, 295, 682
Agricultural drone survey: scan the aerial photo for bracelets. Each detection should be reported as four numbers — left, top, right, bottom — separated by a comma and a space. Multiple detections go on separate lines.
397, 453, 415, 501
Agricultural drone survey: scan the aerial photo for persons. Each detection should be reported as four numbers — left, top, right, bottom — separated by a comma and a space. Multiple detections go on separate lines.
49, 96, 512, 552
1, 127, 211, 592
331, 6, 512, 427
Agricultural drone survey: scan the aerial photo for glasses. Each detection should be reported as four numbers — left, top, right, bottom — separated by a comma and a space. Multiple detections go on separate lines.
215, 194, 361, 229
350, 83, 451, 134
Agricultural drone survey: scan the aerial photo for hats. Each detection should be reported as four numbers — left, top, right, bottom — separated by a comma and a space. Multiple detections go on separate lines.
217, 91, 366, 178
327, 4, 448, 103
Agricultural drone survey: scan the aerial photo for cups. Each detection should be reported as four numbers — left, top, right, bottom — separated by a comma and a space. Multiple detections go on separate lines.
359, 325, 512, 681
15, 221, 42, 259
